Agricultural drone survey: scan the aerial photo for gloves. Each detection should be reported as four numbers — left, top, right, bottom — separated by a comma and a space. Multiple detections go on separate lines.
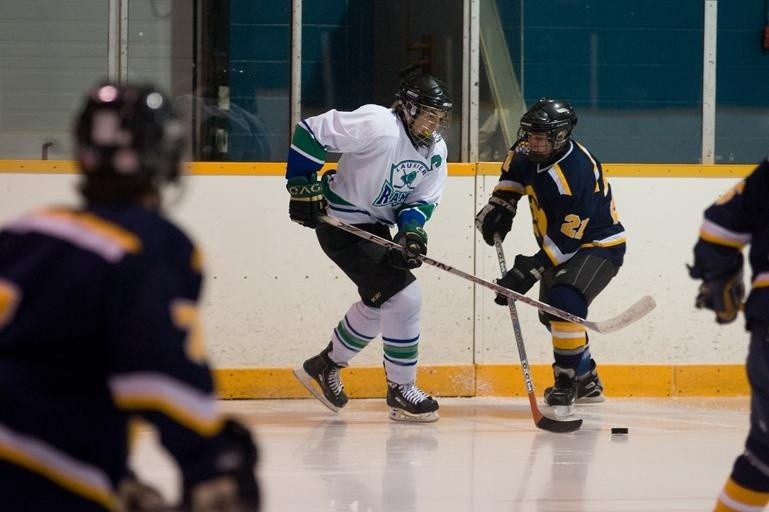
493, 252, 548, 307
474, 189, 522, 247
391, 218, 429, 270
286, 171, 328, 228
699, 252, 746, 326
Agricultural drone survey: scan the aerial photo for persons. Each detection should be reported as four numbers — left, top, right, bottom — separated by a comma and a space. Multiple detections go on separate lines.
285, 75, 456, 412
476, 99, 626, 404
1, 79, 261, 511
687, 156, 769, 511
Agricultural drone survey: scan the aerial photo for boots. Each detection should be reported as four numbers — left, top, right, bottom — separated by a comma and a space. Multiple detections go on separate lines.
383, 359, 440, 414
543, 359, 603, 406
303, 341, 349, 408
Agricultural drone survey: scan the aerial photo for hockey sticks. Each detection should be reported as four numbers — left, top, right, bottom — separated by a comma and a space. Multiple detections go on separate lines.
493, 231, 582, 432
318, 212, 655, 332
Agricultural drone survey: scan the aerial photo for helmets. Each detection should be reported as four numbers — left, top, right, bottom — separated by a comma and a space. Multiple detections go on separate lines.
395, 73, 453, 148
517, 96, 577, 162
203, 67, 259, 114
73, 82, 186, 198
